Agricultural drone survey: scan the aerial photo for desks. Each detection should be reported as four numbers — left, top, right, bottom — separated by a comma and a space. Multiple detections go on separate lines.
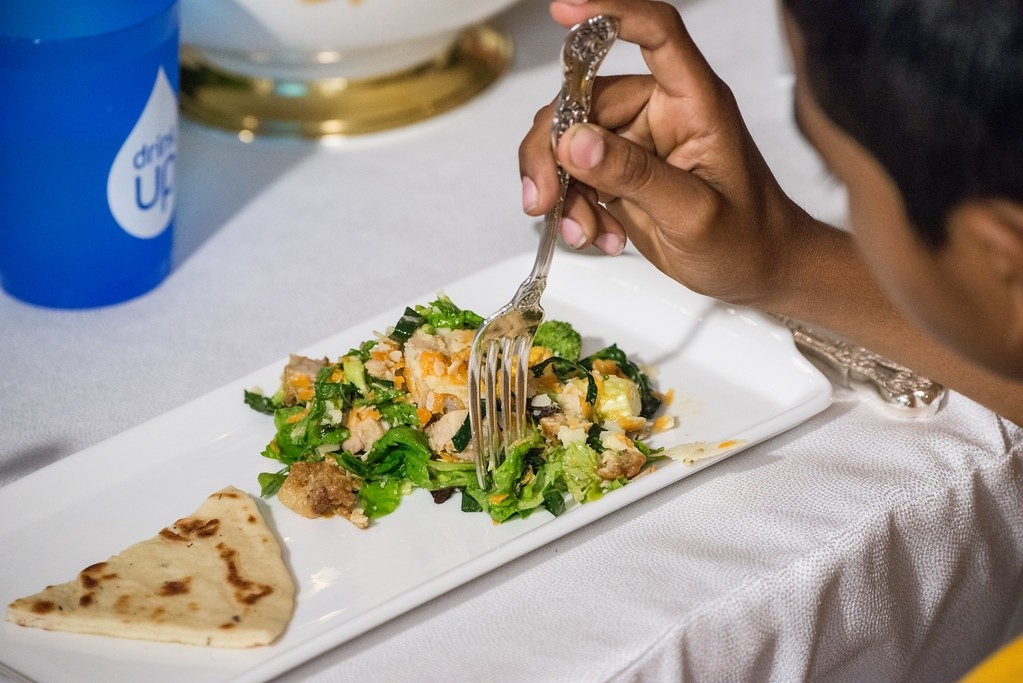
0, 0, 1022, 682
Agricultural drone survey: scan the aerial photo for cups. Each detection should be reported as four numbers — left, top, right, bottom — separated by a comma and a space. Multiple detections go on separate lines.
3, 4, 184, 309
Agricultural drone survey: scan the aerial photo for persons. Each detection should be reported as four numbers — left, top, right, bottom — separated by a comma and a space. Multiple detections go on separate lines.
519, 0, 1023, 428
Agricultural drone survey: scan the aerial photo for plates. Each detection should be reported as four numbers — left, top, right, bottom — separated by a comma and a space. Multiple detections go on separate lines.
1, 245, 835, 679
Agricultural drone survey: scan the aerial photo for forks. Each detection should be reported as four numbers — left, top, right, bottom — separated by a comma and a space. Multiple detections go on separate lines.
467, 13, 621, 487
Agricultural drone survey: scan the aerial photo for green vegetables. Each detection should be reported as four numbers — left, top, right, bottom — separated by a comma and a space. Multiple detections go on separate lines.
240, 294, 666, 521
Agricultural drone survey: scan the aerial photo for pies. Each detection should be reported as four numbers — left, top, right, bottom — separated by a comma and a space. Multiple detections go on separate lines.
8, 484, 296, 647
